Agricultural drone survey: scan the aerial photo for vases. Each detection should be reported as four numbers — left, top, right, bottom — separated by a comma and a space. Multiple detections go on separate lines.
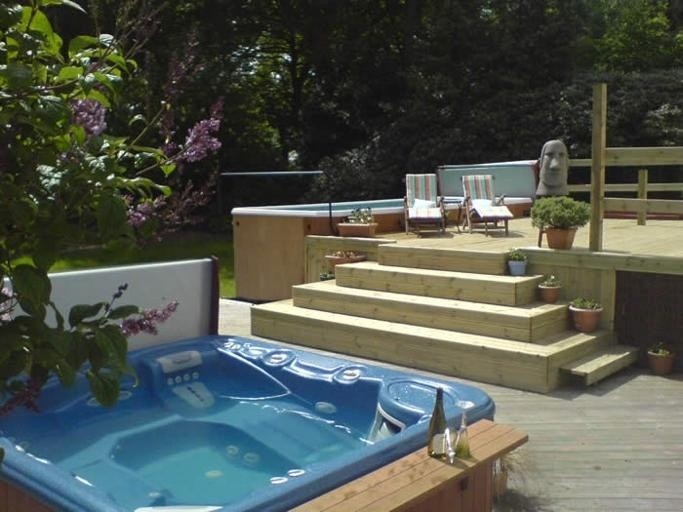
337, 223, 378, 237
325, 254, 367, 275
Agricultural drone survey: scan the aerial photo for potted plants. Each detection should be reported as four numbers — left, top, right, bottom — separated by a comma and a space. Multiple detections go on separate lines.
528, 195, 592, 250
538, 274, 561, 302
568, 295, 603, 331
508, 249, 529, 277
645, 340, 677, 376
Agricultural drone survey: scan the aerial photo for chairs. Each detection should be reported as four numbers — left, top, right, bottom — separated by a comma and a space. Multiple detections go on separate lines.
402, 172, 447, 235
457, 173, 512, 237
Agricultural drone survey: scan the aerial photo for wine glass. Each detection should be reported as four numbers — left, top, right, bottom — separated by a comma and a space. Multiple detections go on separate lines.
452, 400, 475, 459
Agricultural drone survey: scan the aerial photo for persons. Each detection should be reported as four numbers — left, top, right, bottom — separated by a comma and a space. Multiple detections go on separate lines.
535, 139, 568, 195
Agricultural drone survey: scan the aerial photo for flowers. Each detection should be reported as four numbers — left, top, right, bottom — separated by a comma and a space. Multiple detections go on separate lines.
340, 206, 376, 224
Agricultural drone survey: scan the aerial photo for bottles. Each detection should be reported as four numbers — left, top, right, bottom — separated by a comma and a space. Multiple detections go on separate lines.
426, 387, 450, 457
